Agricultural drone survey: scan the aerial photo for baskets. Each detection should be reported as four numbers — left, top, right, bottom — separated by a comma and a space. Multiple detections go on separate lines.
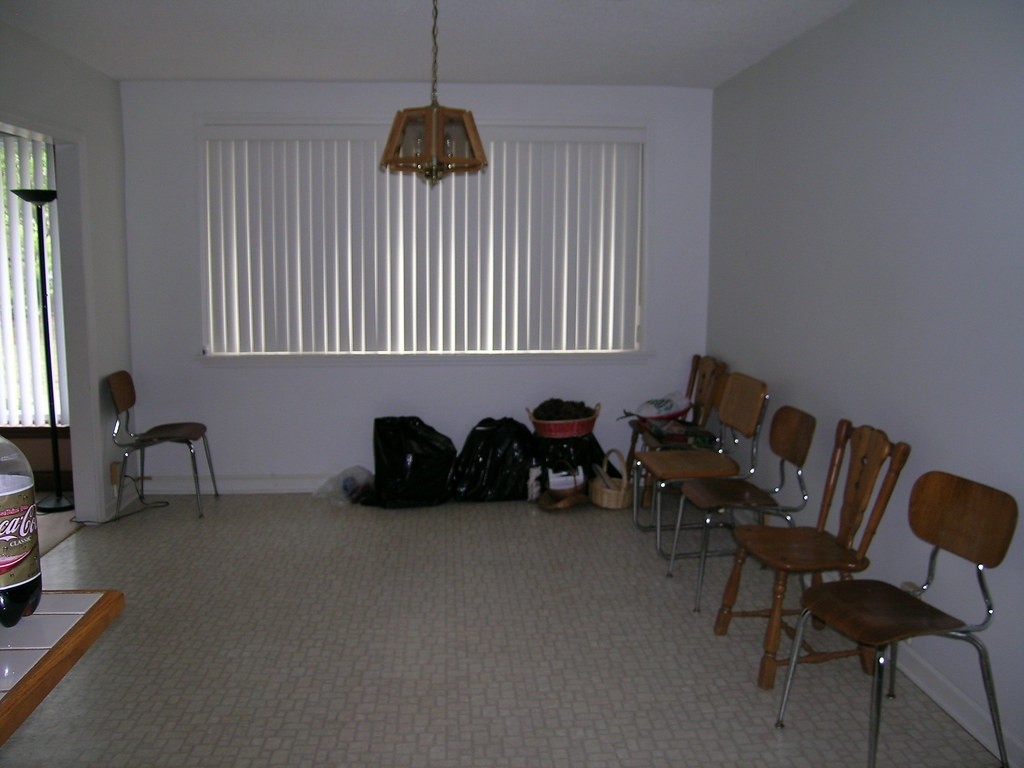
526, 403, 601, 438
590, 449, 632, 509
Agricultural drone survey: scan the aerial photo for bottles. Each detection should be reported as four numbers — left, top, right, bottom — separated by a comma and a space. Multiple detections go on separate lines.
0, 435, 43, 631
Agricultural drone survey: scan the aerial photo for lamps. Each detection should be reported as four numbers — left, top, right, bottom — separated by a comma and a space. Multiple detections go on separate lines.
378, 0, 489, 190
10, 189, 74, 515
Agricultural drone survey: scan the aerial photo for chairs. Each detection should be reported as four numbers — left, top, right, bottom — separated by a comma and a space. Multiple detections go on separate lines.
105, 370, 220, 522
630, 374, 770, 560
714, 419, 911, 691
625, 354, 728, 510
774, 470, 1019, 768
665, 405, 817, 615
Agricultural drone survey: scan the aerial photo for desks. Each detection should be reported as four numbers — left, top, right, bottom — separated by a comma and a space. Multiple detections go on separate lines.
0, 589, 128, 747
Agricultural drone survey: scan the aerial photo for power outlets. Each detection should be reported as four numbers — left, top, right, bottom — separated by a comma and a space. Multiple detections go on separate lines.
111, 461, 122, 485
